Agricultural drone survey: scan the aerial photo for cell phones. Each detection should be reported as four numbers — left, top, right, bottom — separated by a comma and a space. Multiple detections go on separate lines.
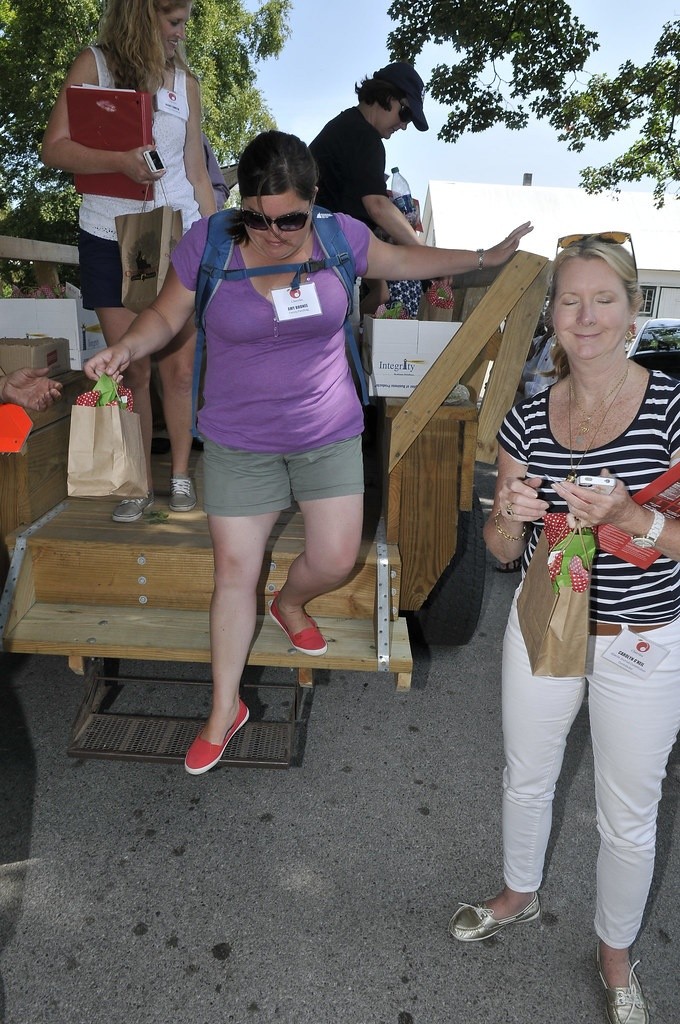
574, 475, 616, 495
143, 149, 166, 172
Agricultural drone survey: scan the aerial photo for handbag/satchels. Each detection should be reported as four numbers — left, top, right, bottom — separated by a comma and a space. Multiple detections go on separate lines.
115, 178, 183, 313
516, 519, 592, 677
66, 375, 148, 501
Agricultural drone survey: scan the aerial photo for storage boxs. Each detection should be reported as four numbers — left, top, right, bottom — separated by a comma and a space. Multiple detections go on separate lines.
0, 297, 109, 377
416, 294, 453, 322
363, 313, 460, 398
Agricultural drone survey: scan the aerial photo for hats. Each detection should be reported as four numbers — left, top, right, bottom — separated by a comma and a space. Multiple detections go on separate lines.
374, 63, 429, 132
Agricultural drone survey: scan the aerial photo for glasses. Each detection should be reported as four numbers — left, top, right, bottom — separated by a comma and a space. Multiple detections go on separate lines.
239, 195, 314, 231
556, 232, 638, 283
396, 93, 412, 124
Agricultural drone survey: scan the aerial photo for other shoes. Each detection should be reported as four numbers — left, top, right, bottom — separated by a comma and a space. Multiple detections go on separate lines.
269, 591, 328, 656
185, 699, 250, 775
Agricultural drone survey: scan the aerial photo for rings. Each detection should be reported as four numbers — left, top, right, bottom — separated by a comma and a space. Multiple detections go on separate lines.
506, 503, 514, 515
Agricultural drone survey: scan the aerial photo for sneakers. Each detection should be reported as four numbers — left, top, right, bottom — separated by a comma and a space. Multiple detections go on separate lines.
112, 490, 154, 522
448, 891, 541, 942
168, 474, 197, 512
595, 939, 649, 1024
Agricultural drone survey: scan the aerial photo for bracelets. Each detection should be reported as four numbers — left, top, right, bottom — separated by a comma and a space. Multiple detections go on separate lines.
493, 513, 527, 541
477, 249, 484, 270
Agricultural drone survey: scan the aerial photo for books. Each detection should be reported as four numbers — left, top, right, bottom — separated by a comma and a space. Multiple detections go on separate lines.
66, 84, 154, 200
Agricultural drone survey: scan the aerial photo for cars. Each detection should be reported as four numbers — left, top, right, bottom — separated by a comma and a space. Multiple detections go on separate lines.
626, 318, 679, 358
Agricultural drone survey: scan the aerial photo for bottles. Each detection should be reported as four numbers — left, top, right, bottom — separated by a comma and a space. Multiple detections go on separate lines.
391, 167, 417, 225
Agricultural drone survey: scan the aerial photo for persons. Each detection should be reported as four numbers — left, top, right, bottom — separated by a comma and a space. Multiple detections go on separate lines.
448, 232, 680, 1024
308, 62, 453, 433
42, 0, 218, 522
518, 302, 561, 398
83, 130, 534, 775
191, 135, 229, 450
360, 212, 423, 317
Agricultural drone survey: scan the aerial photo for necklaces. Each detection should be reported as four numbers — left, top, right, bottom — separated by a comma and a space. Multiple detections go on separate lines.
565, 360, 630, 483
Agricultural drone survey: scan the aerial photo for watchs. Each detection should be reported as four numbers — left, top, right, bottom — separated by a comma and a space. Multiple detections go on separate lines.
629, 505, 665, 549
